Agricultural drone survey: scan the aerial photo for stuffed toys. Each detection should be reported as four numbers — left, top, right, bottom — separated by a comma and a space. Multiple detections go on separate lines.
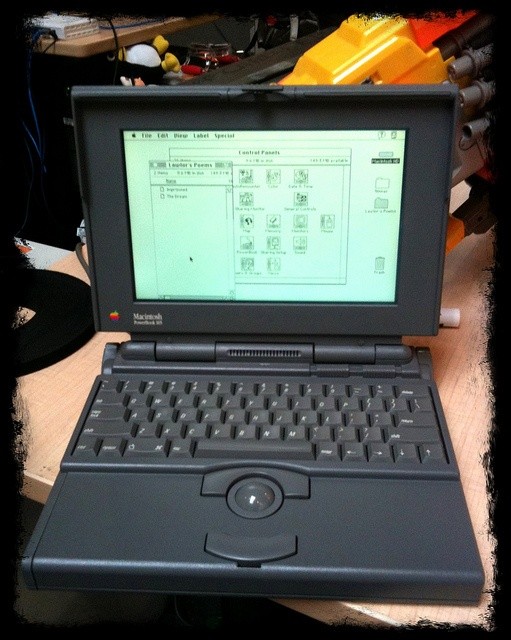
106, 34, 182, 87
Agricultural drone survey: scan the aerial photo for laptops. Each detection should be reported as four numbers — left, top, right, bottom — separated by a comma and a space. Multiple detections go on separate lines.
21, 85, 485, 607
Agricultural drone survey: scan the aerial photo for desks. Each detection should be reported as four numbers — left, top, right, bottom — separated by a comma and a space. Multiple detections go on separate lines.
22, 15, 219, 57
16, 183, 499, 632
